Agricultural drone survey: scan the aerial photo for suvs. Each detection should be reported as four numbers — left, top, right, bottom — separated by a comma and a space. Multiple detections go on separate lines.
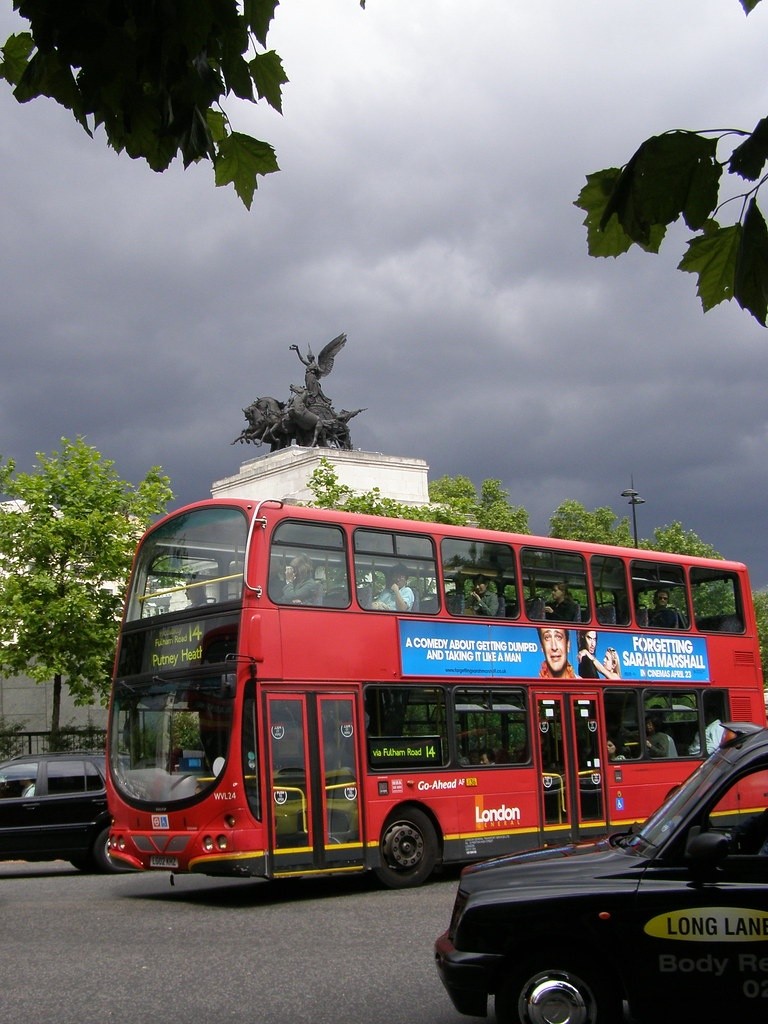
0, 752, 134, 874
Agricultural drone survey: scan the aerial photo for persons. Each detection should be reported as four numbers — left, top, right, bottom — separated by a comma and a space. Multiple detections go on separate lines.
727, 806, 768, 855
577, 629, 622, 679
457, 704, 729, 766
0, 779, 35, 798
289, 344, 332, 405
537, 626, 582, 679
184, 557, 680, 631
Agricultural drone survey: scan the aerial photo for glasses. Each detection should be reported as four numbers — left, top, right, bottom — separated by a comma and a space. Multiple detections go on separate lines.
655, 596, 668, 600
392, 575, 406, 580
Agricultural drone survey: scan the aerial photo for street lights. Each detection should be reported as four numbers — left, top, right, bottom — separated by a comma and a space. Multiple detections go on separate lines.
621, 470, 645, 549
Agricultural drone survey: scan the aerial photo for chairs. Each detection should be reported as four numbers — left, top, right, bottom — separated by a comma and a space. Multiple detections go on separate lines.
324, 585, 685, 628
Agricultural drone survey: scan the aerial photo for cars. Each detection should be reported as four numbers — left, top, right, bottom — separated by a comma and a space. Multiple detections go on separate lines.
435, 723, 768, 1024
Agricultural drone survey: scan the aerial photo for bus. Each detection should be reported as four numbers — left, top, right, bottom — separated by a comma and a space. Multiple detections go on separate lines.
105, 498, 768, 889
